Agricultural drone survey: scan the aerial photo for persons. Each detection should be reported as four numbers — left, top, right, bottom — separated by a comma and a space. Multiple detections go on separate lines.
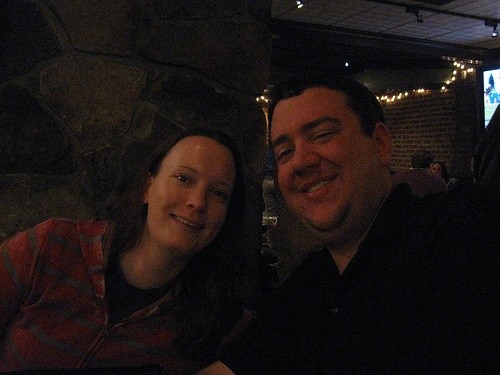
194, 73, 500, 375
0, 125, 254, 375
